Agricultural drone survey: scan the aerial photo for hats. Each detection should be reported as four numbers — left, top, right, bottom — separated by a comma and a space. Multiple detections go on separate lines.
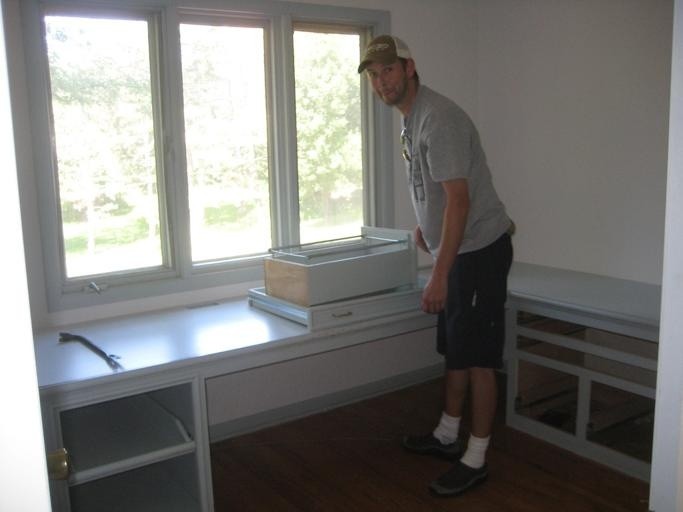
358, 34, 413, 73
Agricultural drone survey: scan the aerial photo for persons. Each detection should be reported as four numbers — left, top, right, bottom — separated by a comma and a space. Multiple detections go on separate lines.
357, 32, 520, 499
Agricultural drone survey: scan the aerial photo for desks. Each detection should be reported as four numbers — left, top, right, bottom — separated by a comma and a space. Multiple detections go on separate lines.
508, 262, 662, 483
37, 269, 445, 508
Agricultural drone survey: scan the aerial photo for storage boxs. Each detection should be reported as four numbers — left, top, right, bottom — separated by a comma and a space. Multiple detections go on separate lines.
247, 225, 429, 331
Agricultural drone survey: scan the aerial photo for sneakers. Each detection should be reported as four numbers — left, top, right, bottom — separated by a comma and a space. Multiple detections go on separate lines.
401, 431, 464, 461
426, 458, 489, 498
